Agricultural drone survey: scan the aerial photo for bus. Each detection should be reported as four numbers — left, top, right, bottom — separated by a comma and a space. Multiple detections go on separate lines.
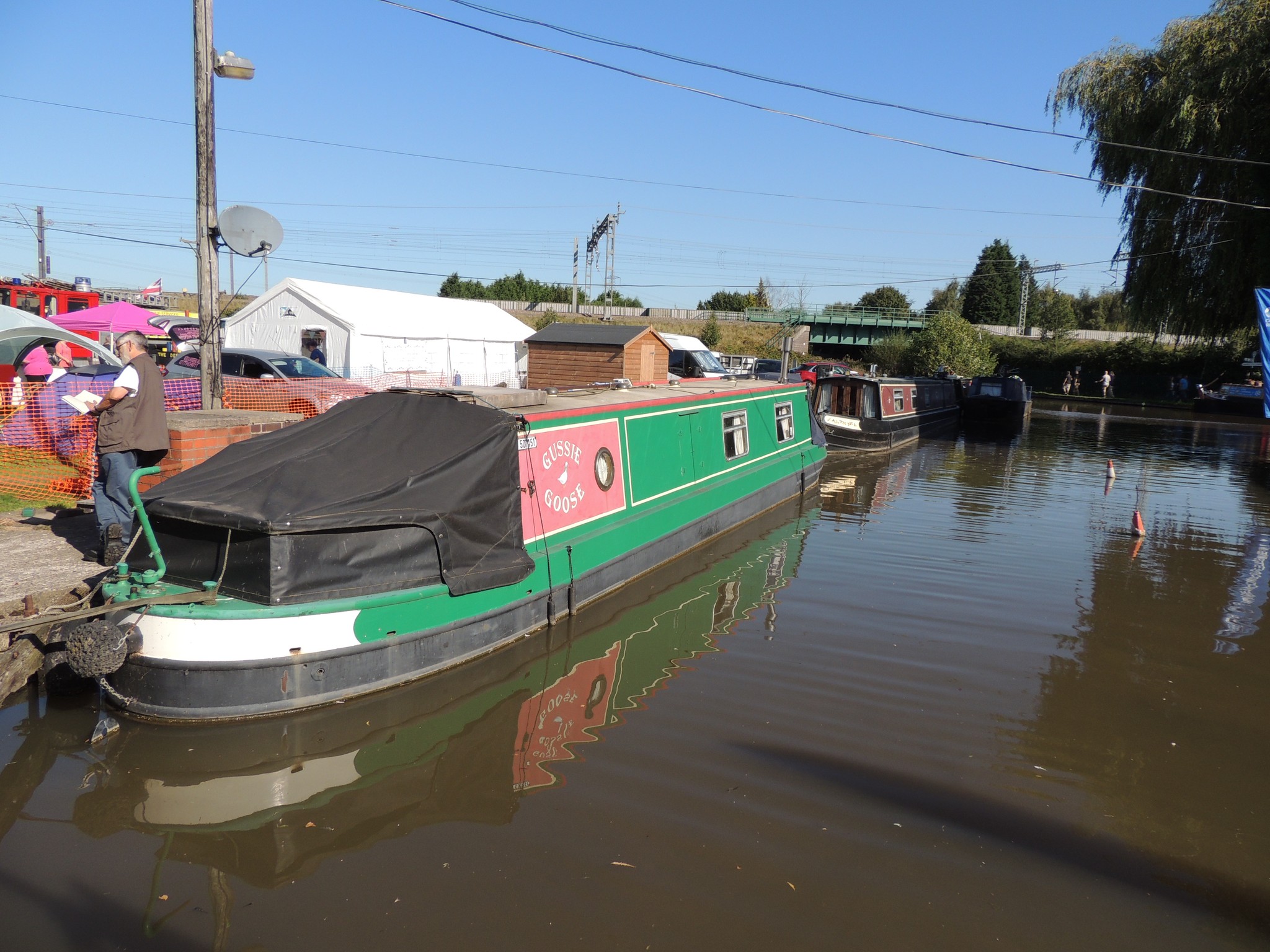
0, 284, 99, 412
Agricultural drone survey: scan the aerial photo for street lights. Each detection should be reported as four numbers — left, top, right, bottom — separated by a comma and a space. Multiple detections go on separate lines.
193, 51, 256, 409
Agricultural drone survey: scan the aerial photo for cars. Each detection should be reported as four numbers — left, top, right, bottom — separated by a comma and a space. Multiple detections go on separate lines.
147, 315, 377, 421
746, 359, 782, 374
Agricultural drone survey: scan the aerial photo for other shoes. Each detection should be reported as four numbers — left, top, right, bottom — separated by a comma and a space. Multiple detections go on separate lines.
83, 548, 104, 561
102, 523, 124, 567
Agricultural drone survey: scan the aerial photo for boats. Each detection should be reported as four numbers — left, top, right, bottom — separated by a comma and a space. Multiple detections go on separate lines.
96, 369, 828, 724
110, 505, 814, 838
964, 373, 1033, 422
810, 373, 960, 453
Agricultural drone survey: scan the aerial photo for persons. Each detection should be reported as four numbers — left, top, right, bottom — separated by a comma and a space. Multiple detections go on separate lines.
1062, 370, 1081, 396
20, 344, 53, 396
1163, 374, 1190, 403
83, 330, 169, 567
1097, 371, 1116, 398
306, 341, 327, 378
54, 340, 74, 367
98, 335, 116, 365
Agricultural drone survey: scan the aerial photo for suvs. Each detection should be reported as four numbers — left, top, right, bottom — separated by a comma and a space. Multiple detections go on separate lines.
789, 361, 864, 391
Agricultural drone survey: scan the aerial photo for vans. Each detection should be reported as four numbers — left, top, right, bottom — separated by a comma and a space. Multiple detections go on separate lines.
657, 332, 735, 380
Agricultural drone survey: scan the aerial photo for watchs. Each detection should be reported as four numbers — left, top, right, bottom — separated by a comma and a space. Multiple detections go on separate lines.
94, 403, 103, 412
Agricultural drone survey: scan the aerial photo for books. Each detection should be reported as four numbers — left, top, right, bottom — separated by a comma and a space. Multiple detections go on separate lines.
61, 390, 103, 414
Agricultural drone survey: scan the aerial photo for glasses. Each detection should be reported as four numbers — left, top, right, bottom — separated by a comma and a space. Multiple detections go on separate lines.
116, 341, 135, 351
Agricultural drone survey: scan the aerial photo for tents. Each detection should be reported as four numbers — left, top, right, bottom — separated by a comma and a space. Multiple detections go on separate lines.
47, 301, 171, 355
